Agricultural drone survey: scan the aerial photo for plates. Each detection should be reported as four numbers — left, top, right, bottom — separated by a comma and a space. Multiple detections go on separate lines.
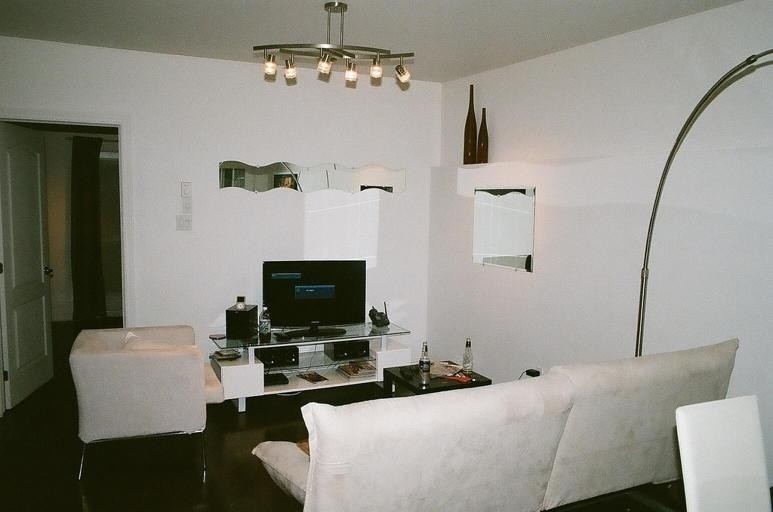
443, 364, 462, 376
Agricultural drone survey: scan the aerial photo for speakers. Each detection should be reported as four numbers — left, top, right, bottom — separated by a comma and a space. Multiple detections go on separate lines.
255, 345, 299, 369
226, 304, 258, 347
324, 339, 369, 362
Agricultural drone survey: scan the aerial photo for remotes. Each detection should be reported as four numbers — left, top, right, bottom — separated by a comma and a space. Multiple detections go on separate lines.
400, 368, 414, 378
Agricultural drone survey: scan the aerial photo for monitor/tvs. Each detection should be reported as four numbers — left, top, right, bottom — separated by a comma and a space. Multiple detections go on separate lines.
262, 260, 366, 338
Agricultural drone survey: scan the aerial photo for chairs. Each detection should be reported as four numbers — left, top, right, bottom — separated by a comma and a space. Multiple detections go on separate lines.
69, 326, 207, 480
676, 395, 772, 512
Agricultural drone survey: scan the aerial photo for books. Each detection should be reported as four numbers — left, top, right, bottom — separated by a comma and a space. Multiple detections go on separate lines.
335, 362, 376, 378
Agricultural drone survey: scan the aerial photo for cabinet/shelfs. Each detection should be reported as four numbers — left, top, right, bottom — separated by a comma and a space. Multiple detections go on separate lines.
210, 322, 412, 413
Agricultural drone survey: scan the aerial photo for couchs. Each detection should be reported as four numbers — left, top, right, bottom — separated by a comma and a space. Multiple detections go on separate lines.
541, 338, 739, 511
252, 372, 576, 512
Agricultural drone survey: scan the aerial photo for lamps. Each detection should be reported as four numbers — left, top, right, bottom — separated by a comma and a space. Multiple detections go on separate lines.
253, 2, 414, 84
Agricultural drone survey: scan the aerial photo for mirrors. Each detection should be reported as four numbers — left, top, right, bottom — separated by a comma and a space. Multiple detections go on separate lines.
218, 160, 406, 195
472, 185, 536, 273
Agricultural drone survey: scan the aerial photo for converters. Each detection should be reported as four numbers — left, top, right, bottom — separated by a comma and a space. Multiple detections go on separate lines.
525, 370, 540, 377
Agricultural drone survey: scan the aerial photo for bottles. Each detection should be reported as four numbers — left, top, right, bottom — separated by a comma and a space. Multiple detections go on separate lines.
463, 338, 473, 375
419, 341, 430, 386
260, 307, 271, 343
